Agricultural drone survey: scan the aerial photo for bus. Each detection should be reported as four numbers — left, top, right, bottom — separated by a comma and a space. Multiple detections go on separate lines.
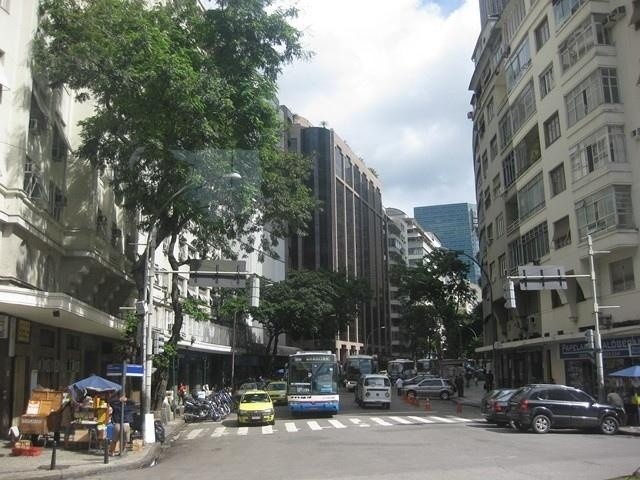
286, 351, 340, 417
387, 358, 476, 385
344, 354, 379, 392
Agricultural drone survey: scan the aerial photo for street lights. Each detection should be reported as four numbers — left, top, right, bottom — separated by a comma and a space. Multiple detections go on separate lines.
138, 173, 242, 428
437, 246, 497, 390
459, 324, 479, 355
366, 326, 385, 354
230, 283, 273, 393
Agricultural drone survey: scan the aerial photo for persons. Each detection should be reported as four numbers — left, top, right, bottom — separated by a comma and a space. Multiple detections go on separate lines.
456, 373, 465, 397
396, 376, 403, 397
474, 366, 481, 386
258, 375, 265, 388
465, 368, 473, 388
607, 386, 623, 407
61, 399, 74, 450
624, 379, 636, 398
178, 382, 187, 406
485, 370, 494, 393
109, 392, 136, 458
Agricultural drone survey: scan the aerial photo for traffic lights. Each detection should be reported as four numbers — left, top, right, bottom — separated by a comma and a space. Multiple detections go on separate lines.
503, 281, 515, 309
154, 328, 164, 354
584, 330, 594, 350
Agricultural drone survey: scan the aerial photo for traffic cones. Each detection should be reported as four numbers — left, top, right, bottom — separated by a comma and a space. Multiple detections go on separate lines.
425, 397, 432, 411
456, 400, 462, 416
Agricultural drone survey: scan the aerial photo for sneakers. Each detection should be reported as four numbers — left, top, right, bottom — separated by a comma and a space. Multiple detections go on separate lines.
108, 452, 126, 456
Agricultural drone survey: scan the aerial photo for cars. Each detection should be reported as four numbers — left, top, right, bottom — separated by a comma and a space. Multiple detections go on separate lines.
235, 382, 288, 426
479, 384, 627, 434
398, 375, 455, 400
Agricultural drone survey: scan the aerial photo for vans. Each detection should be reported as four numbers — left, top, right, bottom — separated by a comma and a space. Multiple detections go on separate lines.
355, 374, 392, 409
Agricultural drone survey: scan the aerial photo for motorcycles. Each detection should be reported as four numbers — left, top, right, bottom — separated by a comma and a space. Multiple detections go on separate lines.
129, 413, 165, 443
184, 383, 234, 422
